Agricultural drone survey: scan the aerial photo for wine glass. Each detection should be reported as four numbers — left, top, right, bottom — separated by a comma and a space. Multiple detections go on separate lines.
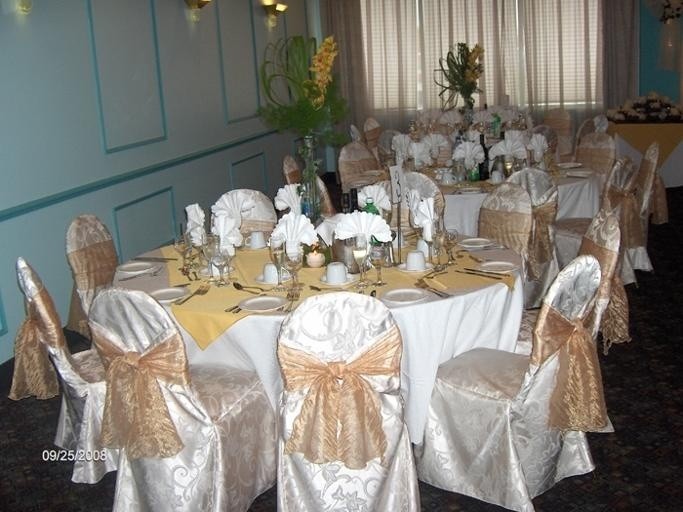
174, 229, 459, 292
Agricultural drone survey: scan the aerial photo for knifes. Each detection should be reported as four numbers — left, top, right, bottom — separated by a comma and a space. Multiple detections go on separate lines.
131, 257, 178, 262
227, 293, 267, 312
455, 267, 510, 280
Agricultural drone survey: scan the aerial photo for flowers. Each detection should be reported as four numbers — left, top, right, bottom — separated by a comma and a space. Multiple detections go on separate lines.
605, 91, 682, 120
258, 34, 350, 148
432, 42, 485, 110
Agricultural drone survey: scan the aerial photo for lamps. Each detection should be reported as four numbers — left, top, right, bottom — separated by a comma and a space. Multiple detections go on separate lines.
261, 0, 288, 28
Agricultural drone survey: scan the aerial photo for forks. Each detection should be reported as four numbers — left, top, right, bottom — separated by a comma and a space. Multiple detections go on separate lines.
119, 266, 162, 281
171, 285, 211, 307
416, 279, 452, 297
284, 289, 301, 312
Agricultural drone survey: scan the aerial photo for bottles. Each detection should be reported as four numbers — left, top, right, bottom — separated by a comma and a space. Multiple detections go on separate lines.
467, 131, 512, 182
302, 180, 379, 217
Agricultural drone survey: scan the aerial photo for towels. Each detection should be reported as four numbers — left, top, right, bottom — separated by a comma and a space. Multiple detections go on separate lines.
184, 203, 208, 247
267, 212, 369, 258
274, 184, 307, 213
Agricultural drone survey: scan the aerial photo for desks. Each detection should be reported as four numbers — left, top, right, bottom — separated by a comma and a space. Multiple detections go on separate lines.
607, 121, 683, 187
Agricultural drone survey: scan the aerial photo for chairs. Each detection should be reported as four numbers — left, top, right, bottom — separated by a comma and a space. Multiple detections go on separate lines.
64, 212, 120, 343
274, 291, 422, 512
209, 188, 279, 233
412, 255, 614, 512
8, 256, 118, 484
86, 288, 278, 511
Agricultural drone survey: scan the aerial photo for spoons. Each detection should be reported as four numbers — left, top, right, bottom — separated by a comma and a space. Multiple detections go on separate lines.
233, 283, 266, 294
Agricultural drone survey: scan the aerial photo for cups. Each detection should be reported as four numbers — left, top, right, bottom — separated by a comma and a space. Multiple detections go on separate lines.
245, 231, 429, 283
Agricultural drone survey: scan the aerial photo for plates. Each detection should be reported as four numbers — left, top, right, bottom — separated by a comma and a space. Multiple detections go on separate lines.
121, 261, 152, 275
457, 162, 593, 194
240, 297, 289, 313
472, 262, 517, 274
381, 289, 427, 303
148, 287, 191, 303
460, 238, 493, 250
